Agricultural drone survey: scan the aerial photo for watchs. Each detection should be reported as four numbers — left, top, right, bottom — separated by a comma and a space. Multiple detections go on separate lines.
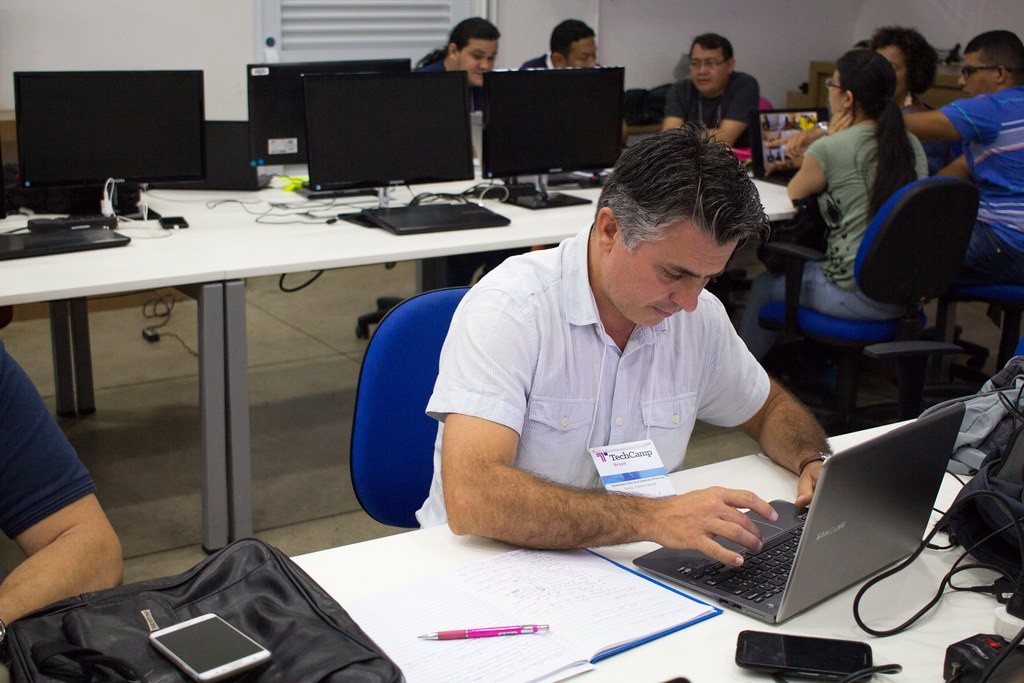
798, 451, 831, 476
0, 619, 8, 646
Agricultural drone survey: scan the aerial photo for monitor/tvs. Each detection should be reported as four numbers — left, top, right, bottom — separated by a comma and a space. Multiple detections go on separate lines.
247, 59, 626, 229
13, 70, 207, 223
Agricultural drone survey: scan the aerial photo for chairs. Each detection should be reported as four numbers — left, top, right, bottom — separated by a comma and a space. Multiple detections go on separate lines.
757, 176, 1024, 438
350, 286, 470, 529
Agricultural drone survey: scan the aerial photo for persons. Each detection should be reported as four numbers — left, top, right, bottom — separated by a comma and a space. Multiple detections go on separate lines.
658, 34, 764, 181
783, 113, 801, 129
415, 17, 501, 113
739, 47, 927, 360
414, 122, 832, 566
871, 24, 949, 174
0, 344, 121, 649
518, 18, 600, 71
763, 30, 1024, 284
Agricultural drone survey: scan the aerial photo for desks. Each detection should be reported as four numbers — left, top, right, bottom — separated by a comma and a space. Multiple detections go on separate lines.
289, 418, 1024, 683
0, 159, 797, 556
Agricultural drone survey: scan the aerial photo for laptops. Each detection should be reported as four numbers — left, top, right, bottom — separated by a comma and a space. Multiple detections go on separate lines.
752, 106, 830, 186
631, 401, 967, 625
362, 204, 511, 235
0, 226, 131, 260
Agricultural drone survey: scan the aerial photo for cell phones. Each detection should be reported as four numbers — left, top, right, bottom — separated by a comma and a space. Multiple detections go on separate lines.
735, 630, 874, 683
159, 216, 189, 229
149, 611, 273, 683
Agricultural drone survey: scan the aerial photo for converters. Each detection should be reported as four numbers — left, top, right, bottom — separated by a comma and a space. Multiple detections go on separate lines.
143, 329, 160, 342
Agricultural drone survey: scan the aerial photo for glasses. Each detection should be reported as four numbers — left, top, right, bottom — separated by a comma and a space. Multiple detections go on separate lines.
959, 64, 1013, 78
824, 76, 848, 94
688, 57, 731, 70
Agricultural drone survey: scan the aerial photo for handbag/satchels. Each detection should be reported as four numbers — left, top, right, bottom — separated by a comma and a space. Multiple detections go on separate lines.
0, 536, 402, 683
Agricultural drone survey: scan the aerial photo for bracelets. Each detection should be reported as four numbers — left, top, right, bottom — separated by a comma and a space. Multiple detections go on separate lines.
816, 121, 829, 135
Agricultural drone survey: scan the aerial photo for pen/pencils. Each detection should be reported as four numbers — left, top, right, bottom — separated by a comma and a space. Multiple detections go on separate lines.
417, 624, 550, 640
